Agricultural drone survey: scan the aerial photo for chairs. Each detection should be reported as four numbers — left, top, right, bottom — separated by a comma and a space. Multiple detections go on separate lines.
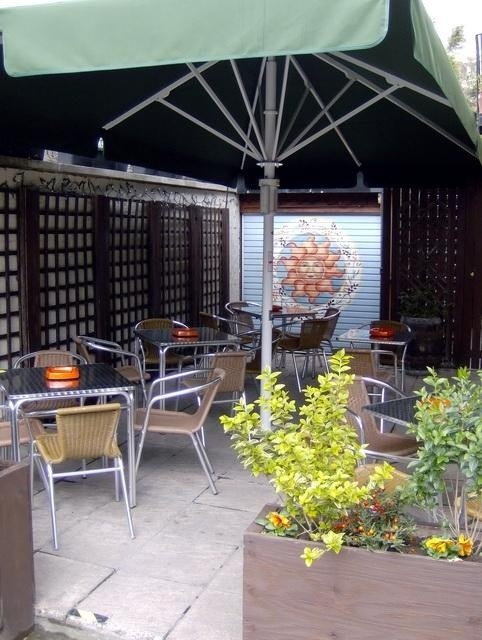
70, 291, 343, 417
132, 366, 226, 496
337, 320, 458, 502
21, 404, 136, 551
0, 351, 88, 480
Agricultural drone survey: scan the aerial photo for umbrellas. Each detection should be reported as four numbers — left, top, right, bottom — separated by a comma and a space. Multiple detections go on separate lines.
0, 1, 482, 434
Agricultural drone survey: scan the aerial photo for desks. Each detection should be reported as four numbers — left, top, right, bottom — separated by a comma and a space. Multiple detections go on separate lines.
1, 361, 136, 509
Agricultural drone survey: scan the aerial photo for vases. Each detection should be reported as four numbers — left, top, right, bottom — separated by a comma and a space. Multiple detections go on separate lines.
245, 503, 482, 640
